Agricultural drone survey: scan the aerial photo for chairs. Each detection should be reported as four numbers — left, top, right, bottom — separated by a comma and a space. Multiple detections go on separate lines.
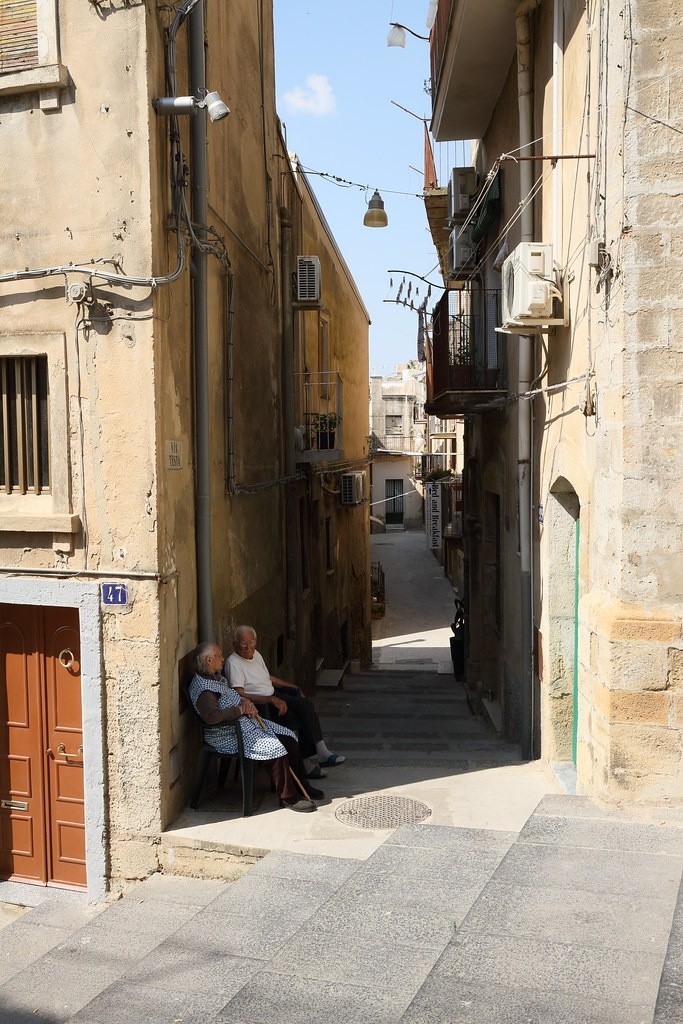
181, 673, 286, 816
245, 687, 302, 721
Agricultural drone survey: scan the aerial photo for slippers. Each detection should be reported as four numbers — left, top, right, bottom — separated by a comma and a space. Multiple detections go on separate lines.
303, 765, 329, 779
317, 754, 347, 767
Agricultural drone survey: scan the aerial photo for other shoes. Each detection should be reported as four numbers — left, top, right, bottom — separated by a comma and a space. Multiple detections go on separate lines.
278, 794, 316, 813
296, 783, 324, 801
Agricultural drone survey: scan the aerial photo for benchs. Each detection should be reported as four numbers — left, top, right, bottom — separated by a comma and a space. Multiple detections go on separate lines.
315, 656, 344, 691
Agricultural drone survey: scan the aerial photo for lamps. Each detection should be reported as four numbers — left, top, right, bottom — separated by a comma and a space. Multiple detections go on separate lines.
363, 189, 388, 227
387, 23, 406, 47
154, 86, 231, 123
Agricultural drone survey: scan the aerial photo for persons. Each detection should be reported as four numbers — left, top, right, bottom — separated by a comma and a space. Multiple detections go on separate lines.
225, 625, 346, 780
189, 642, 324, 813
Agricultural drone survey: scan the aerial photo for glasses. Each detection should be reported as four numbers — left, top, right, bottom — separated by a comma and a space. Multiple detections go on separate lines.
235, 639, 256, 646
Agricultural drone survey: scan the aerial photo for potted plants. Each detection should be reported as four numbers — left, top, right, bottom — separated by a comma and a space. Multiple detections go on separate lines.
451, 343, 475, 380
313, 412, 342, 450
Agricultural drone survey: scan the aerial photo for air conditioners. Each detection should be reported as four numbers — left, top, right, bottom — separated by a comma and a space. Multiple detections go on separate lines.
447, 167, 477, 228
342, 473, 362, 505
449, 225, 476, 281
502, 241, 552, 327
297, 255, 321, 300
347, 471, 367, 501
